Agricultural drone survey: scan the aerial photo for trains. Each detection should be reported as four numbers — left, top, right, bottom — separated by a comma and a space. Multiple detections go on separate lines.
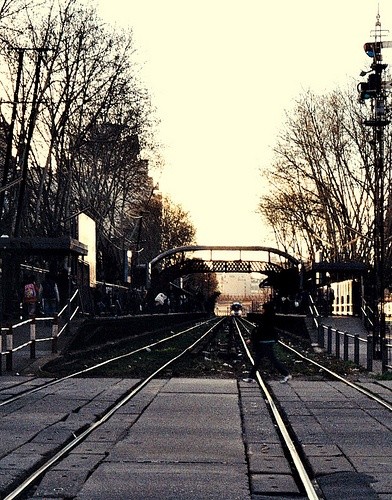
229, 303, 243, 316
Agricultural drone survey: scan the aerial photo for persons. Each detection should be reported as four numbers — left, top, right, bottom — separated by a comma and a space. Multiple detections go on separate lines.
23, 275, 39, 318
95, 286, 143, 317
242, 303, 293, 384
39, 272, 60, 327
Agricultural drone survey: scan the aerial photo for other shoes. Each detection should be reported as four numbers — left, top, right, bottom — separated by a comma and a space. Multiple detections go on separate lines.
29, 313, 35, 315
279, 374, 292, 383
243, 377, 256, 383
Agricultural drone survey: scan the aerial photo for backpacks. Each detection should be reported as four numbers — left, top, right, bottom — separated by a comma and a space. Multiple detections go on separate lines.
24, 284, 35, 298
